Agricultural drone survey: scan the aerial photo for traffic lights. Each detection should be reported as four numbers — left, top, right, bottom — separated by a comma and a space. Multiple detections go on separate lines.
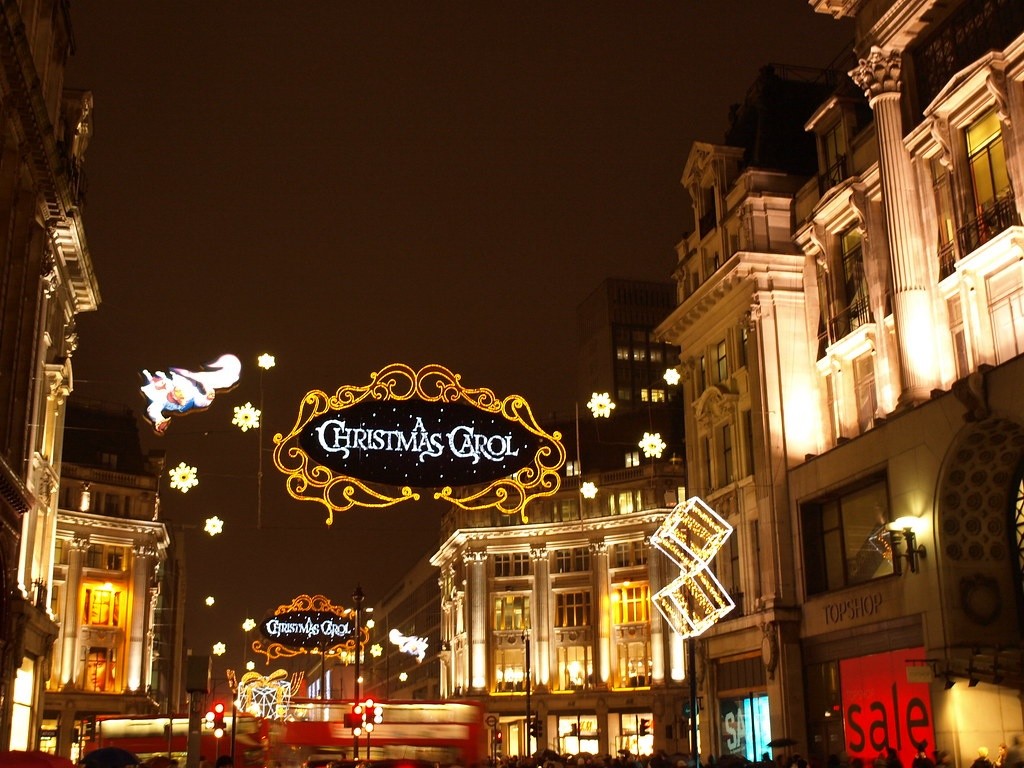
85, 714, 98, 743
537, 719, 544, 737
530, 718, 537, 737
496, 730, 503, 744
570, 723, 576, 736
641, 715, 650, 736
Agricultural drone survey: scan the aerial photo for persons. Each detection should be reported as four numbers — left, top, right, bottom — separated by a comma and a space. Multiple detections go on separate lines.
427, 747, 692, 768
699, 752, 864, 768
89, 646, 116, 692
80, 748, 233, 768
872, 736, 1024, 768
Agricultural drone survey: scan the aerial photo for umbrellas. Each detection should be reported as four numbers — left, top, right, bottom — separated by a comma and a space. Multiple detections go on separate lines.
767, 738, 799, 753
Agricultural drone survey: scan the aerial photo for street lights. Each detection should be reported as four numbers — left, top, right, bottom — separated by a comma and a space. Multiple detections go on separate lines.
351, 581, 366, 760
